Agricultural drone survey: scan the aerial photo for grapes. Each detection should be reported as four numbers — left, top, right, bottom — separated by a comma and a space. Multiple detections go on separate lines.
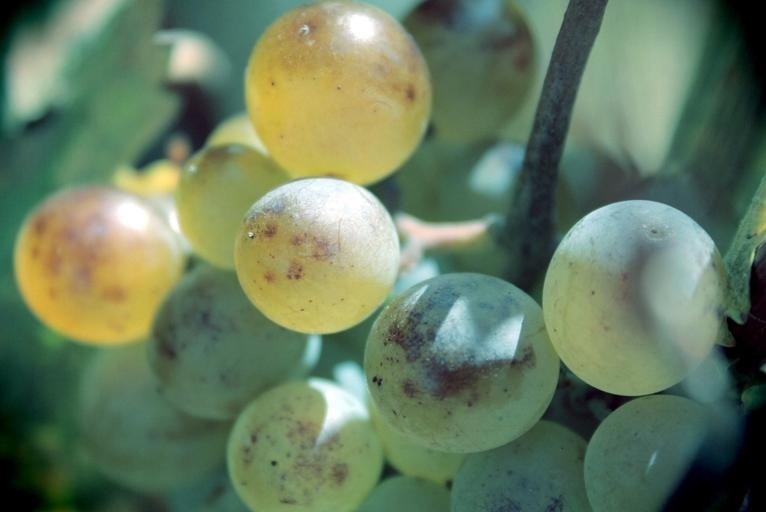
12, 0, 745, 512
